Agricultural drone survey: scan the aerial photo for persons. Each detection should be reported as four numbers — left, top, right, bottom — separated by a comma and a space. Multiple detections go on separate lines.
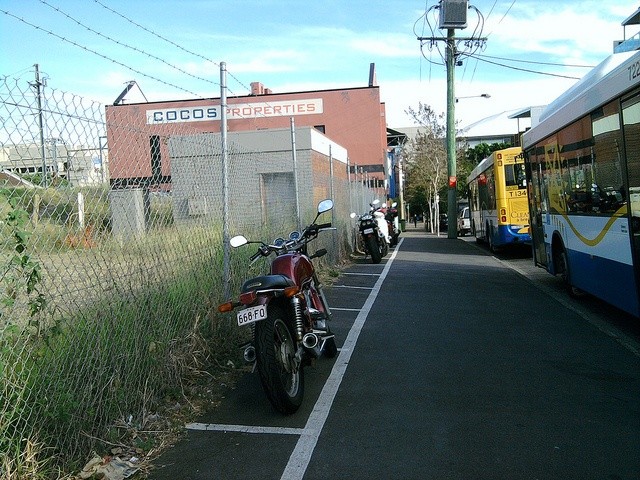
413, 214, 417, 228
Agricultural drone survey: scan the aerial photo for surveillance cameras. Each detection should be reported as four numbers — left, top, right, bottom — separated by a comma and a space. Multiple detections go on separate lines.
454, 97, 459, 104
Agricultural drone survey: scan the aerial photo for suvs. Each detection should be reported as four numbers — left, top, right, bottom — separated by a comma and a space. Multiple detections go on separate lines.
439, 214, 448, 229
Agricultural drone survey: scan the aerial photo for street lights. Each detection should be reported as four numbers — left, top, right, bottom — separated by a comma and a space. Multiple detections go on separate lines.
452, 94, 492, 103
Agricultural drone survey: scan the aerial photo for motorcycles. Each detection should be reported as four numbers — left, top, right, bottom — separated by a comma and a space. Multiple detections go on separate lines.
380, 202, 401, 246
218, 198, 337, 417
350, 199, 388, 263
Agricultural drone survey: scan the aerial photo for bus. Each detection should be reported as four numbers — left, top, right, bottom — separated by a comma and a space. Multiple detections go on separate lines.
520, 32, 639, 322
462, 146, 533, 252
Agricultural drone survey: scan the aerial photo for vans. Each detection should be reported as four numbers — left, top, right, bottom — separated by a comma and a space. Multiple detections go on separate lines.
457, 206, 471, 236
